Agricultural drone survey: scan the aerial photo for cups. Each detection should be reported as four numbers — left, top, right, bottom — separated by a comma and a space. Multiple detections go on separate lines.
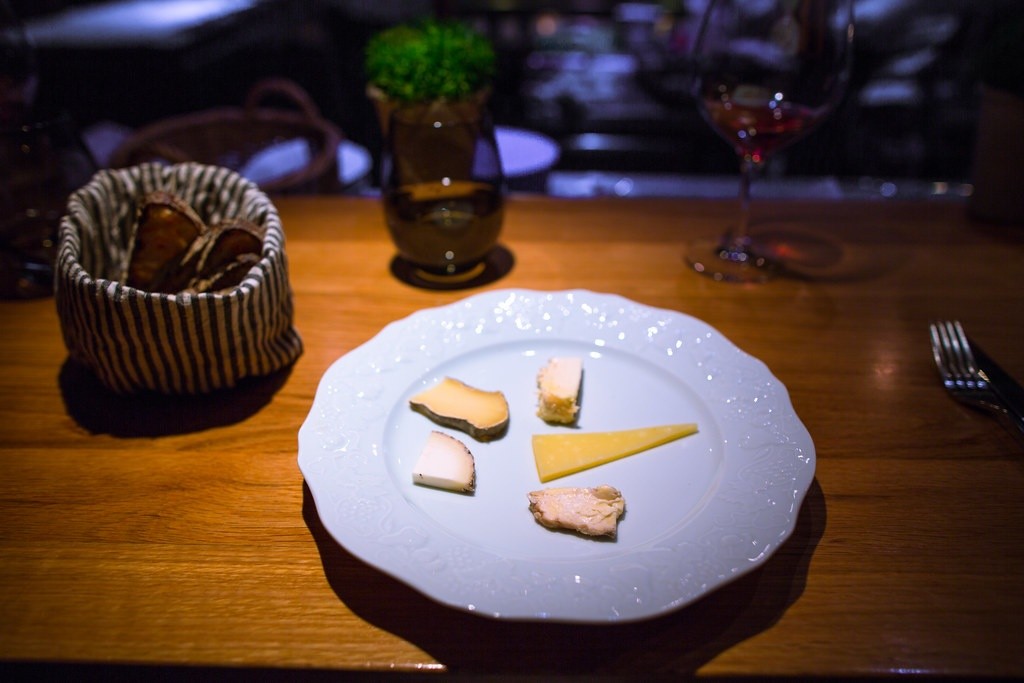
382, 105, 503, 270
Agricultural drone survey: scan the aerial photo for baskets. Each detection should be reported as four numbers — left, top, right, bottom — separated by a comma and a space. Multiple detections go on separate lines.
109, 78, 341, 196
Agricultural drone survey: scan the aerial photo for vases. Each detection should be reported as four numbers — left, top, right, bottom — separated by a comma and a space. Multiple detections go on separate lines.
364, 70, 507, 288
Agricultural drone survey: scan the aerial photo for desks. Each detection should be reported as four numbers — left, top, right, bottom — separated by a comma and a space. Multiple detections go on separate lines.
1, 185, 1024, 683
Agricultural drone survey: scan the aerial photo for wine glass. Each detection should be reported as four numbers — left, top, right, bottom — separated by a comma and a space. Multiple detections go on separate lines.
683, 1, 851, 280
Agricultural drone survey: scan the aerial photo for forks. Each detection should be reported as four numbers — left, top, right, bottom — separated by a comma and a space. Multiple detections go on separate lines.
929, 322, 1024, 446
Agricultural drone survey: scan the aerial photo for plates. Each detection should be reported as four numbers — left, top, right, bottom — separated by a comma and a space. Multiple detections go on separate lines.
297, 291, 815, 622
